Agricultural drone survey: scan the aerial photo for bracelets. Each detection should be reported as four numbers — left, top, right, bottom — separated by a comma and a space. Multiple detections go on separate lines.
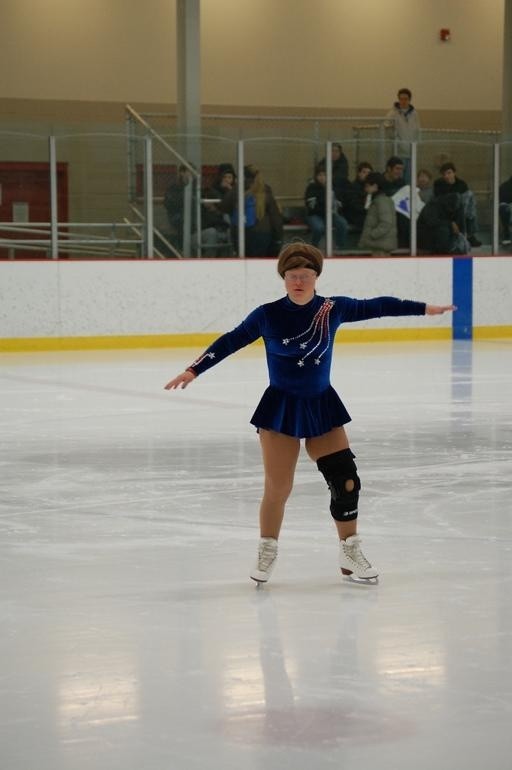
185, 369, 198, 378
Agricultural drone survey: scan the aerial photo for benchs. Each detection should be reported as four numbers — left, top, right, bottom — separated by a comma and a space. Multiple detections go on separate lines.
277, 196, 359, 233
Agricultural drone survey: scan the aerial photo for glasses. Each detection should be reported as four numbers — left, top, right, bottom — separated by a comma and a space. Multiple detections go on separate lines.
284, 271, 318, 282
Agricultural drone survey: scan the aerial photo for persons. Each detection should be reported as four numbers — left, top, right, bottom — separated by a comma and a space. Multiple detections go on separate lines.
381, 88, 420, 171
164, 243, 459, 596
323, 143, 351, 186
500, 175, 511, 245
303, 156, 484, 256
162, 162, 284, 258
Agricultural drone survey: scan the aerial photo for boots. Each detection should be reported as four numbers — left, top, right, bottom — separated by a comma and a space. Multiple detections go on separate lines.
339, 535, 379, 579
249, 536, 279, 583
